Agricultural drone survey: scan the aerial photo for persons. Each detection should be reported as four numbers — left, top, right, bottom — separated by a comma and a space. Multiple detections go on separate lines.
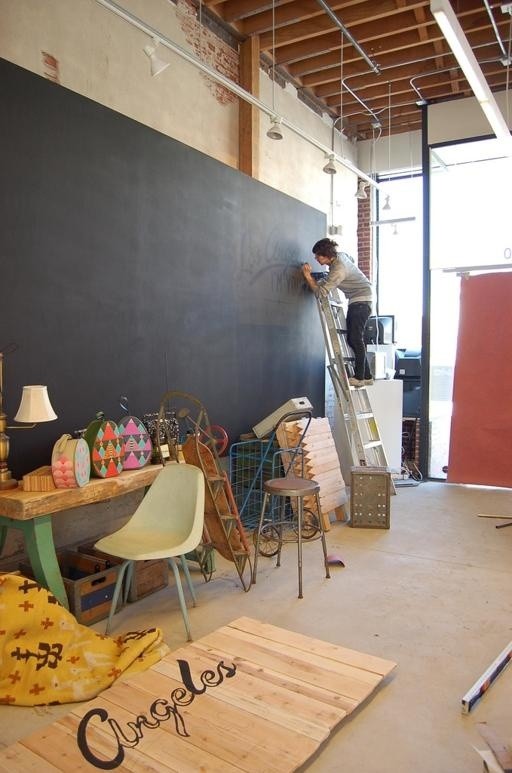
303, 239, 374, 386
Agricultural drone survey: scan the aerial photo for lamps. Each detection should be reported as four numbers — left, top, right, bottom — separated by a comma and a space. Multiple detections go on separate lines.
0, 385, 58, 490
95, 0, 392, 211
429, 0, 512, 156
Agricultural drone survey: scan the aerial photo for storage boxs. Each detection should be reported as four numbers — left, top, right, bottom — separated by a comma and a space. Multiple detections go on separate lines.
18, 550, 123, 626
69, 534, 169, 602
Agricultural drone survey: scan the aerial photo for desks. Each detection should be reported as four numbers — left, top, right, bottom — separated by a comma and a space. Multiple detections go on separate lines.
333, 379, 404, 486
0, 460, 216, 612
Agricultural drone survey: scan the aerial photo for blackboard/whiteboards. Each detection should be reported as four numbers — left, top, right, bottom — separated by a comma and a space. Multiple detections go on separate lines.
0, 616, 396, 773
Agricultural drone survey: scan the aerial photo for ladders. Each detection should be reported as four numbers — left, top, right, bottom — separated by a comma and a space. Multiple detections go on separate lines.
310, 272, 397, 496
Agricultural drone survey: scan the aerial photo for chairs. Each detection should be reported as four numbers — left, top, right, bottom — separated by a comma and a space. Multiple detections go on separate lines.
94, 463, 206, 642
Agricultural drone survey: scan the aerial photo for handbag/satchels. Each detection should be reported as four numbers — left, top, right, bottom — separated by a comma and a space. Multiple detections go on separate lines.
112, 415, 152, 470
84, 419, 125, 479
51, 436, 91, 488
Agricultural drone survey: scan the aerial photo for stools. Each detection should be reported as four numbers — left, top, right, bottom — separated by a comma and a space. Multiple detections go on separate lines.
250, 478, 331, 599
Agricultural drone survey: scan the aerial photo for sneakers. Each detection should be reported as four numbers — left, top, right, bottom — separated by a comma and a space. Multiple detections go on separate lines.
348, 377, 374, 387
